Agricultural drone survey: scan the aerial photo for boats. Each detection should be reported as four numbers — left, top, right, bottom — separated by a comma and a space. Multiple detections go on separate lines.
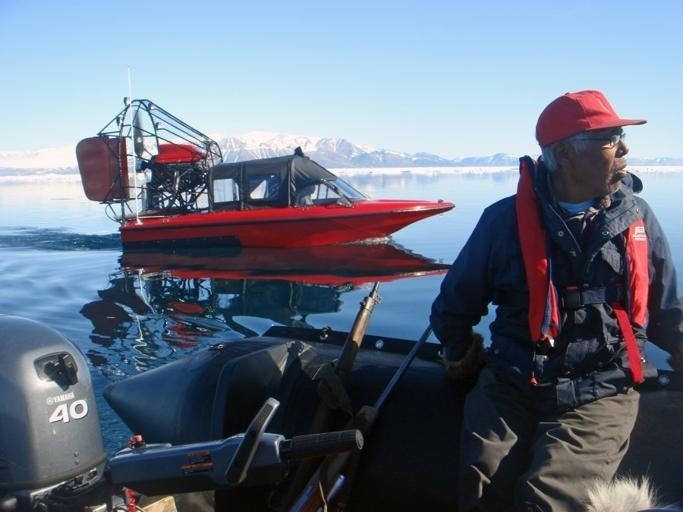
0, 303, 682, 512
70, 97, 456, 251
81, 246, 454, 388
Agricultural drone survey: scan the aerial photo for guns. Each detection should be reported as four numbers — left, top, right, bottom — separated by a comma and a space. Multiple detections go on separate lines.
267, 280, 433, 512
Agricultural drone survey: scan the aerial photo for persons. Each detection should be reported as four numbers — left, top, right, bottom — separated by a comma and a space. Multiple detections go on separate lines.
297, 184, 315, 206
430, 88, 683, 512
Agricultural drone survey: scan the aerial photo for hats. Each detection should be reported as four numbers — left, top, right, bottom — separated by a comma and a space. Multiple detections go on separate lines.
535, 90, 646, 149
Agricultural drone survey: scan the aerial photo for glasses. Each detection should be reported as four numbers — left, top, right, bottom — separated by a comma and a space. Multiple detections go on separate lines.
575, 131, 625, 152
